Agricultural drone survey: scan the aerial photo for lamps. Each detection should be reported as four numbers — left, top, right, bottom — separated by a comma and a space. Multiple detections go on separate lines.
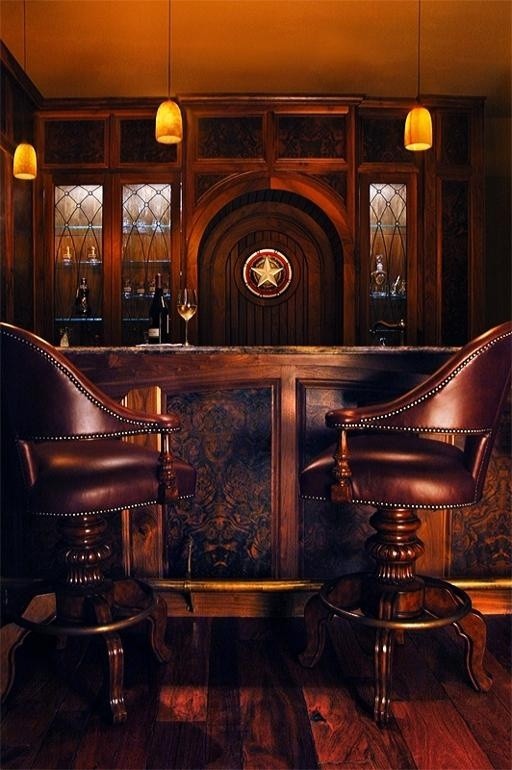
12, 0, 38, 180
404, 0, 433, 152
155, 0, 184, 144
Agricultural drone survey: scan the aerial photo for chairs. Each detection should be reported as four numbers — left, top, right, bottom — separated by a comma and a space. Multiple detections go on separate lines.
1, 321, 198, 724
298, 320, 512, 724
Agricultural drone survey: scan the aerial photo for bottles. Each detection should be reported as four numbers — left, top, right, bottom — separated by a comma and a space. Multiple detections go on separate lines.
77, 278, 90, 318
369, 253, 389, 297
120, 273, 170, 343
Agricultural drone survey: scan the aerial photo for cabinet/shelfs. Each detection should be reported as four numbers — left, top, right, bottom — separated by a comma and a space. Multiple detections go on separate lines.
357, 169, 423, 346
41, 170, 182, 345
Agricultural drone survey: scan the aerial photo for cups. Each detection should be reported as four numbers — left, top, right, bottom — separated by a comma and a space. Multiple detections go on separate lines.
62, 184, 167, 266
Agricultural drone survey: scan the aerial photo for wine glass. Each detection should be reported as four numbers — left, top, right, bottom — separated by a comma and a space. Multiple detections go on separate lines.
174, 287, 199, 346
